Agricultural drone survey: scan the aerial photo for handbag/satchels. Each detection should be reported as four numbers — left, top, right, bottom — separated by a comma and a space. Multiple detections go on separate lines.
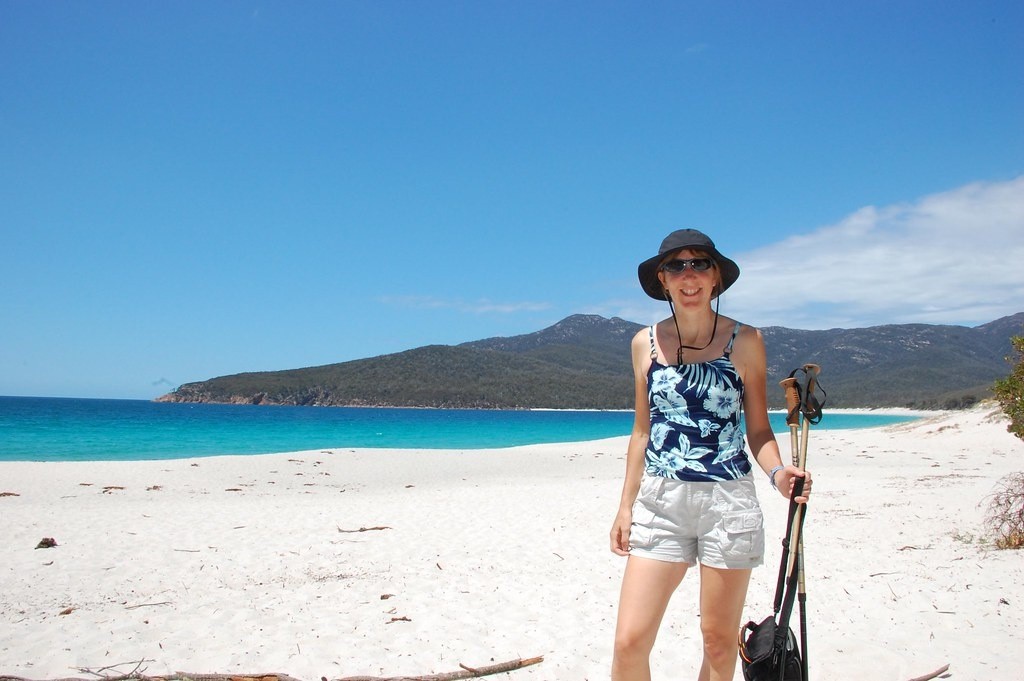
736, 613, 803, 681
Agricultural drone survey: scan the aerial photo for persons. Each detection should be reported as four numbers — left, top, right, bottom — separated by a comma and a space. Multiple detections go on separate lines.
610, 228, 813, 681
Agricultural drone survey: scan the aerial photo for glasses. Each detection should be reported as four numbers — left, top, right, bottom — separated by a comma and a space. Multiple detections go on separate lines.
655, 256, 716, 274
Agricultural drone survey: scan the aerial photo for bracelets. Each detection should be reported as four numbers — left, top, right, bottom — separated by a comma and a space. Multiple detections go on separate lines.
770, 466, 783, 490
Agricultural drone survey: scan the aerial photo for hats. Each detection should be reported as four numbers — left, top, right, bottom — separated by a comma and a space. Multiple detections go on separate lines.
637, 229, 740, 302
